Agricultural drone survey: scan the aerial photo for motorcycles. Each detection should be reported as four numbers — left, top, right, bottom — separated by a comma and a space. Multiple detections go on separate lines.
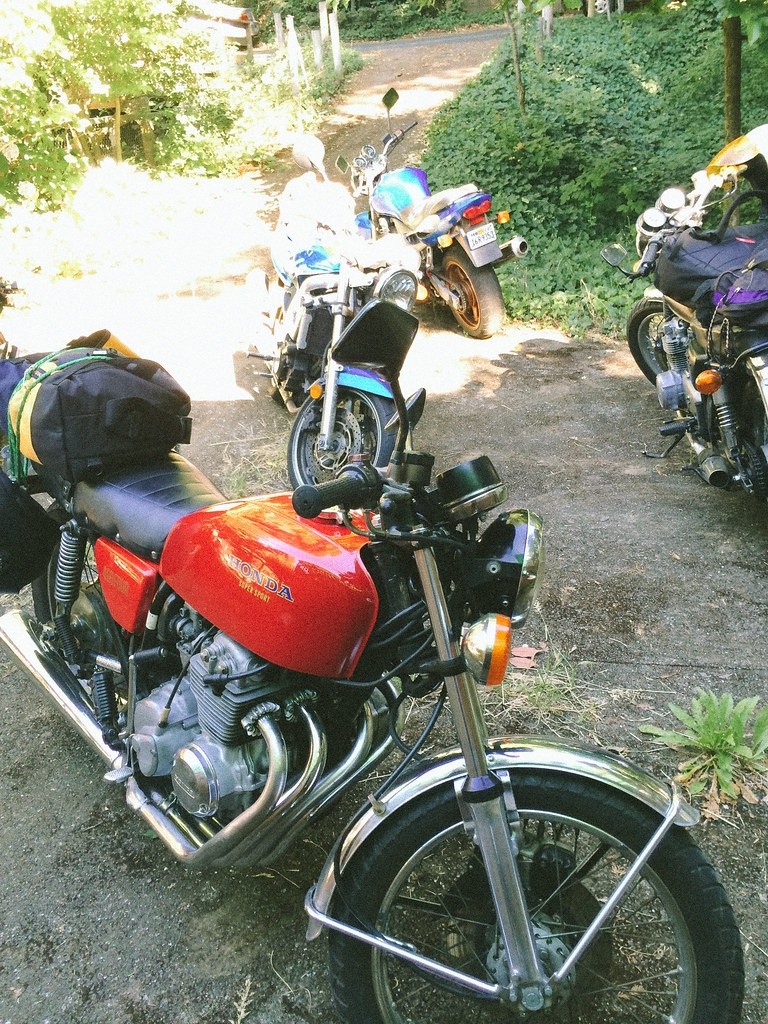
335, 87, 529, 340
0, 298, 745, 1024
246, 135, 424, 490
600, 165, 768, 501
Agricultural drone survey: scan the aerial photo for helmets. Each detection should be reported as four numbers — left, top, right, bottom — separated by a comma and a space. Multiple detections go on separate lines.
706, 123, 768, 195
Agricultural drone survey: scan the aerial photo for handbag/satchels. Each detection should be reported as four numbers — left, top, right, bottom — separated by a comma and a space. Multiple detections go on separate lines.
690, 237, 768, 328
0, 468, 64, 594
0, 329, 193, 487
